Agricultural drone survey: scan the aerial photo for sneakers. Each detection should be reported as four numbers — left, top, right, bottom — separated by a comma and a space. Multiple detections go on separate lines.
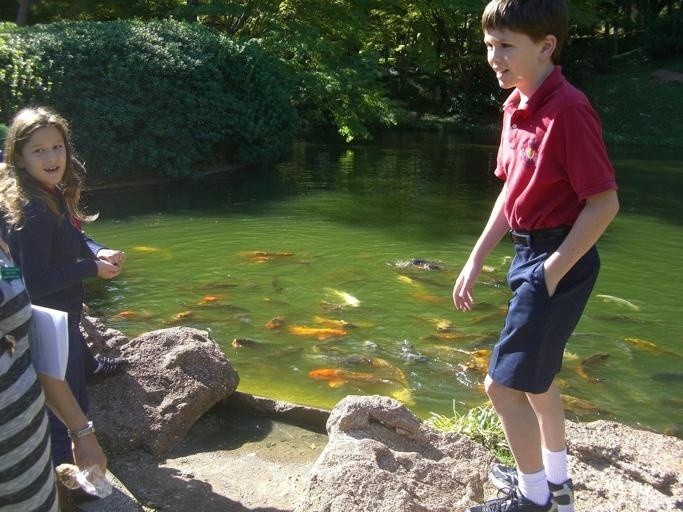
467, 462, 575, 512
86, 355, 129, 386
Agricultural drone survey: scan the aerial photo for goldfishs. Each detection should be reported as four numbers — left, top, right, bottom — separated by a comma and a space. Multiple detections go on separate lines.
158, 250, 644, 426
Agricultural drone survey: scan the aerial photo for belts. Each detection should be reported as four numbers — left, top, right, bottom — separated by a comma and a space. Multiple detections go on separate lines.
507, 224, 571, 247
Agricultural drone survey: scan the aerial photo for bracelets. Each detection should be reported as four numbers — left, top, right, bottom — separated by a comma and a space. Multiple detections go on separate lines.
65, 420, 95, 441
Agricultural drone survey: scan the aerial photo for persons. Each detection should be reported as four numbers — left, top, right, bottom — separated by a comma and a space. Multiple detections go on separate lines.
450, 0, 622, 512
0, 104, 127, 470
0, 144, 109, 512
